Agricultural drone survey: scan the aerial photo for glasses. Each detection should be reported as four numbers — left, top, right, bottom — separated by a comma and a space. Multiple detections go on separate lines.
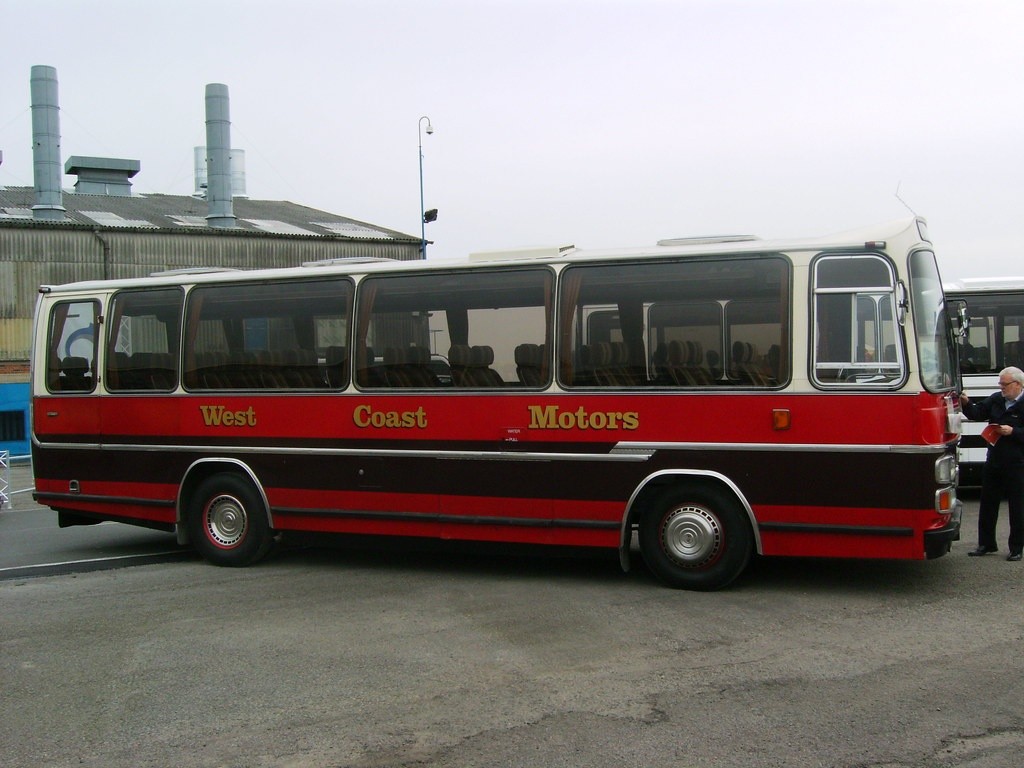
998, 380, 1016, 386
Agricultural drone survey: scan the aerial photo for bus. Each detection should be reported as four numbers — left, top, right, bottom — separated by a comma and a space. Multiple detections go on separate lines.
28, 215, 970, 591
563, 276, 1024, 489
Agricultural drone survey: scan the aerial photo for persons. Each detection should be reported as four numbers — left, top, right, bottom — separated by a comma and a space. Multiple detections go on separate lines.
960, 366, 1024, 560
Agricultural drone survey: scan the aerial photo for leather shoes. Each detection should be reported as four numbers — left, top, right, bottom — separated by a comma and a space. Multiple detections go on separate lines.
1007, 550, 1022, 561
968, 544, 998, 556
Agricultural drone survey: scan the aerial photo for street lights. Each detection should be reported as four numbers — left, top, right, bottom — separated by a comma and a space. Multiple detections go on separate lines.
418, 116, 435, 260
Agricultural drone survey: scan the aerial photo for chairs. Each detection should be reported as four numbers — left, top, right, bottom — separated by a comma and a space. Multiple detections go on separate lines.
115, 349, 324, 390
382, 345, 446, 387
325, 346, 381, 387
652, 339, 714, 385
731, 340, 780, 386
586, 341, 638, 389
448, 345, 504, 389
515, 343, 548, 387
